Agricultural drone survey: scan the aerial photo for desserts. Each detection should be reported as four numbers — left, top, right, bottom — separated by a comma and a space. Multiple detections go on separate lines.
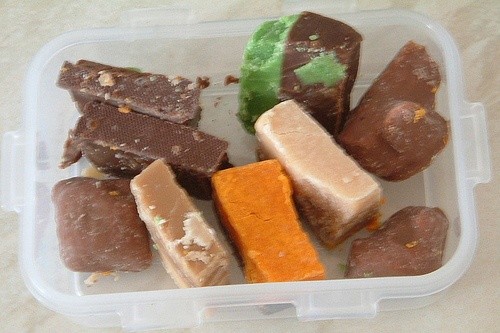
52, 11, 449, 287
